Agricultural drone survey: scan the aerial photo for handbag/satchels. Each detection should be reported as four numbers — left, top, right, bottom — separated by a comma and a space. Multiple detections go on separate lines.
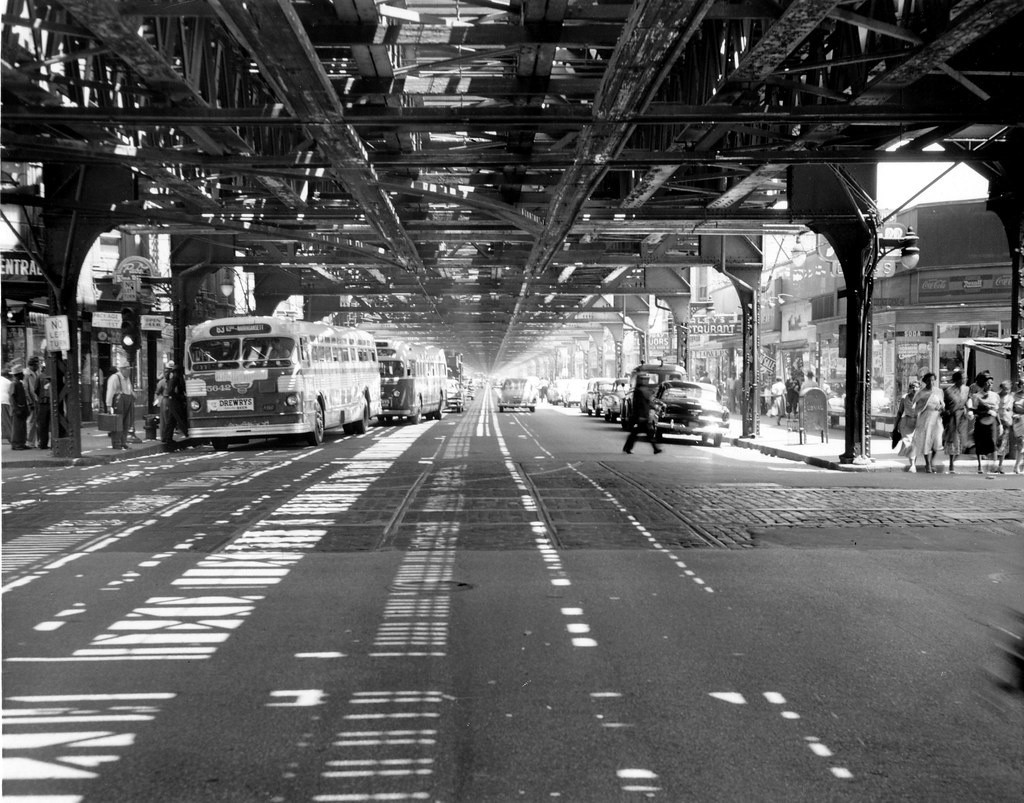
766, 404, 776, 418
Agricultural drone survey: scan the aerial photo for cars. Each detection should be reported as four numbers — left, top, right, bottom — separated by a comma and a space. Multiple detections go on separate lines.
649, 380, 730, 448
546, 356, 687, 430
497, 377, 539, 414
444, 349, 487, 414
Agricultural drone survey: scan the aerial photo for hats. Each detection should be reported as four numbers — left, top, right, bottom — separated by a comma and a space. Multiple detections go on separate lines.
165, 360, 175, 368
805, 370, 813, 375
27, 356, 40, 365
116, 358, 129, 367
8, 364, 24, 374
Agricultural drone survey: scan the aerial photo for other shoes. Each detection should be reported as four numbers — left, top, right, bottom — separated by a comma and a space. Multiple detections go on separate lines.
11, 446, 24, 450
40, 445, 50, 449
166, 439, 177, 445
121, 442, 132, 449
624, 448, 630, 453
113, 444, 123, 450
161, 438, 166, 442
653, 448, 661, 453
20, 445, 31, 449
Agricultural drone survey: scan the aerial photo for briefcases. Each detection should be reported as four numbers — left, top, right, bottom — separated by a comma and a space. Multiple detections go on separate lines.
97, 413, 123, 432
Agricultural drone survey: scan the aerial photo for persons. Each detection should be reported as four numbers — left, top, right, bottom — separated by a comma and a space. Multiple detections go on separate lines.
700, 371, 743, 415
892, 369, 1024, 474
539, 378, 549, 402
393, 362, 404, 377
623, 376, 667, 455
99, 360, 191, 450
1, 357, 65, 450
765, 369, 820, 426
267, 340, 291, 366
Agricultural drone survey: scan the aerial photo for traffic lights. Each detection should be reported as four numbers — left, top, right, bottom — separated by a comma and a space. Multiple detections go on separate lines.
120, 301, 141, 352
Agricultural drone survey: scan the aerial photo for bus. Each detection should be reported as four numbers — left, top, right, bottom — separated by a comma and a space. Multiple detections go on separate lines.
183, 315, 382, 451
375, 339, 448, 423
